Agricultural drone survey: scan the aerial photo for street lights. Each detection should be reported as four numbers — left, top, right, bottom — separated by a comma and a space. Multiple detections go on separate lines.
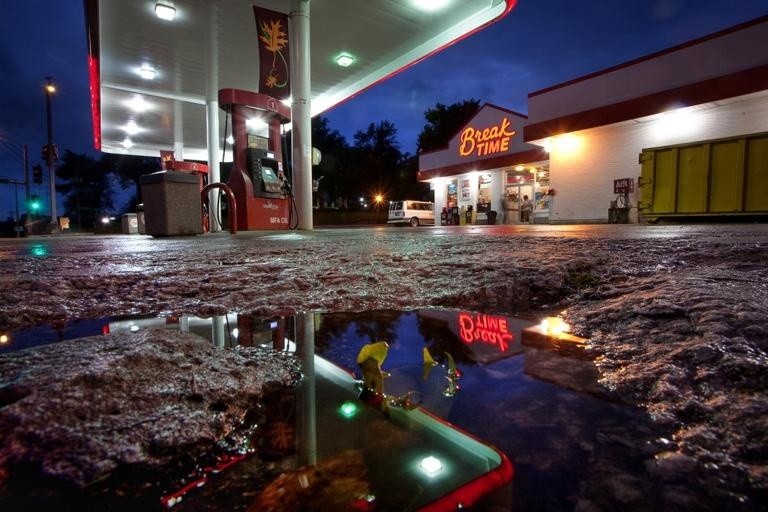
42, 74, 62, 236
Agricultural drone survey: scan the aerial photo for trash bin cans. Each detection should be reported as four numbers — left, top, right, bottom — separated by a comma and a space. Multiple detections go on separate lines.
486, 211, 497, 225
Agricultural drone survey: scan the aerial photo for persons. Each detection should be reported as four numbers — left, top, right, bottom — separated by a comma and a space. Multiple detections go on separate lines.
521, 195, 533, 222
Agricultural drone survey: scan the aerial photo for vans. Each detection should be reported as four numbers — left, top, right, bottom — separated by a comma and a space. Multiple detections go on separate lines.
385, 199, 434, 227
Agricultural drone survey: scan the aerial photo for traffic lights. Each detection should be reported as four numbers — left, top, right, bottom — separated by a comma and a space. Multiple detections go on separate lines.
40, 144, 49, 160
32, 162, 41, 184
30, 193, 39, 213
